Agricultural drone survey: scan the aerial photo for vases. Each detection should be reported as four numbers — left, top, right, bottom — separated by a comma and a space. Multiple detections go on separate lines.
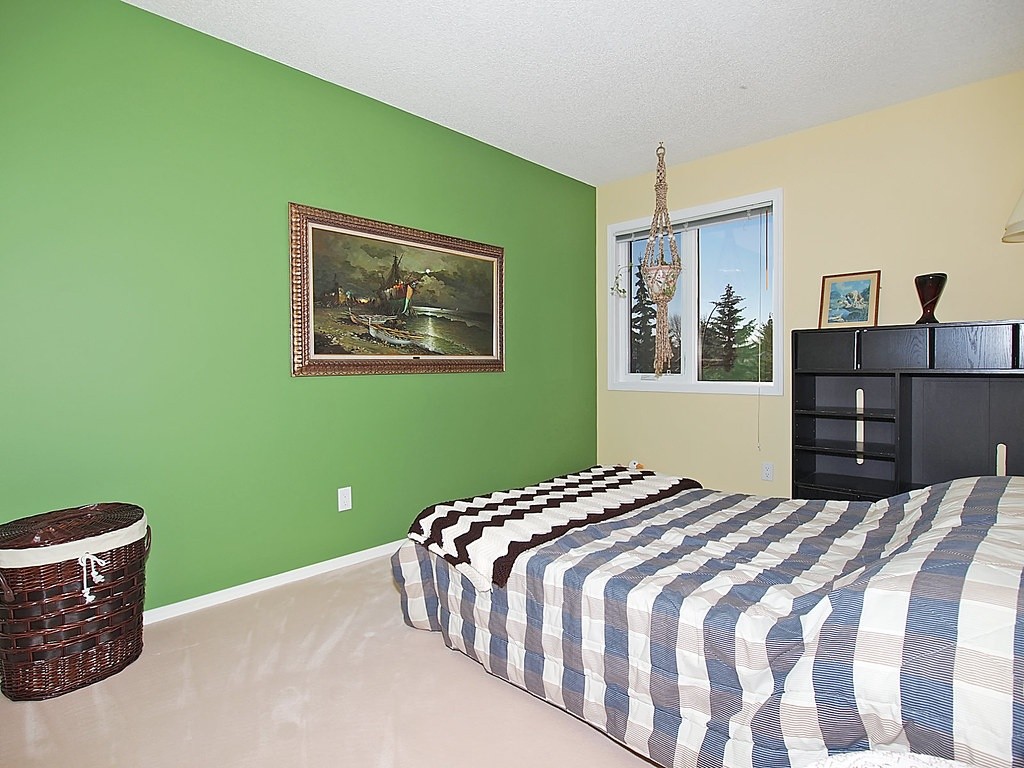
915, 272, 947, 325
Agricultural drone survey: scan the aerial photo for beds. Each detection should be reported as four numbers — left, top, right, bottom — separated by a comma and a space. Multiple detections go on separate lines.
391, 465, 1024, 768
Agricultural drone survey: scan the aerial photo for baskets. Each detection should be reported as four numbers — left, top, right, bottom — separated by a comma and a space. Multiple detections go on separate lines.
2, 503, 150, 702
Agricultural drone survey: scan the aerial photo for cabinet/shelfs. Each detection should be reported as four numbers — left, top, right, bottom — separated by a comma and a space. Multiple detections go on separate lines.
791, 320, 1024, 503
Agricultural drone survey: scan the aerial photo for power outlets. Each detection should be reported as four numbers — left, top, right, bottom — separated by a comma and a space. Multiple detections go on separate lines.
338, 486, 352, 512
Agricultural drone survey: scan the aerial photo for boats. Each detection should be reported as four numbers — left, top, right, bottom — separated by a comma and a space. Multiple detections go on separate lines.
368, 318, 412, 346
347, 309, 397, 328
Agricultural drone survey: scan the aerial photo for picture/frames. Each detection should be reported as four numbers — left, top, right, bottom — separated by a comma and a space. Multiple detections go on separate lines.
817, 269, 881, 330
286, 200, 506, 378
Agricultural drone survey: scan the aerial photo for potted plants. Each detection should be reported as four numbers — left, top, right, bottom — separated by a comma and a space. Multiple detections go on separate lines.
610, 250, 682, 304
